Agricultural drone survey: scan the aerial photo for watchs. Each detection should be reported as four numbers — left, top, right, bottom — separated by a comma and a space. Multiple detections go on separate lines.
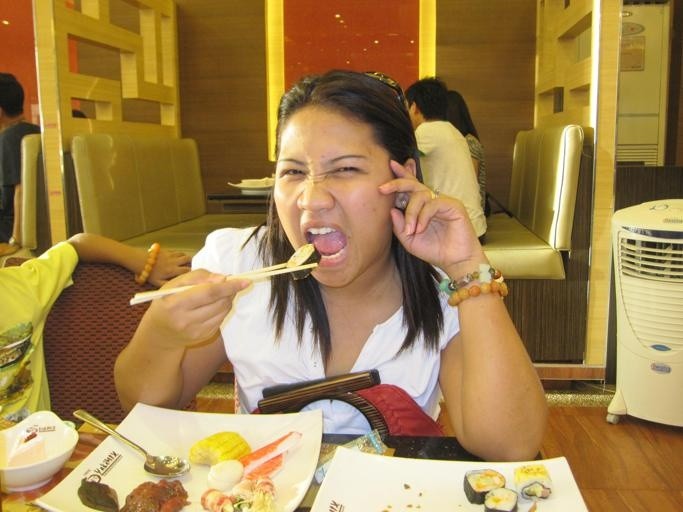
8, 238, 21, 249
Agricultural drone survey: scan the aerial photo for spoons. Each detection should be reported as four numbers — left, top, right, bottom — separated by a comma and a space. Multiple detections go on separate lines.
72, 406, 191, 477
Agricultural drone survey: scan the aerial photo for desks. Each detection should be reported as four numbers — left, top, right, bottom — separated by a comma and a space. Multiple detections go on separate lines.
204, 189, 273, 212
0, 413, 545, 512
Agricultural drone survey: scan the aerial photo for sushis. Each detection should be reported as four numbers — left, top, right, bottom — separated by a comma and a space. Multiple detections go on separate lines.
464, 468, 506, 505
485, 488, 520, 512
287, 244, 322, 280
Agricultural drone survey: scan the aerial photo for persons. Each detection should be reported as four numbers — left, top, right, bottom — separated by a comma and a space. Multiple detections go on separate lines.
0, 232, 193, 425
114, 69, 549, 462
405, 78, 488, 244
446, 91, 487, 215
0, 72, 40, 258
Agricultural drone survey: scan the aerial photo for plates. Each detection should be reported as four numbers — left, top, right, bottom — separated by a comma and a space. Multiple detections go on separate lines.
306, 444, 588, 512
227, 182, 274, 196
31, 400, 324, 511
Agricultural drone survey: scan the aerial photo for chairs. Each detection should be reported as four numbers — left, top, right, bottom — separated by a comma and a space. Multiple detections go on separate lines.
6, 256, 199, 416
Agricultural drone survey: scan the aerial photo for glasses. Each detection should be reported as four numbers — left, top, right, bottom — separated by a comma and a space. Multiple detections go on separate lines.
364, 70, 407, 106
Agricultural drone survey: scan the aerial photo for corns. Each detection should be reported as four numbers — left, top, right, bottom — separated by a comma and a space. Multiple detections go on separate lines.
188, 431, 251, 468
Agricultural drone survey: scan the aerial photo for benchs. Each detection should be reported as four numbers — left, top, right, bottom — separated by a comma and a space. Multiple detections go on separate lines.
75, 137, 268, 263
478, 124, 593, 361
1, 131, 51, 256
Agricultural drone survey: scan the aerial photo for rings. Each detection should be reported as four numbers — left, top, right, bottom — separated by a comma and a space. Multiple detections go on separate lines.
430, 184, 440, 198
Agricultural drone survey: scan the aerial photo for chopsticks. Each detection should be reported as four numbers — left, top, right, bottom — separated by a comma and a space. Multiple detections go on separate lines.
130, 260, 317, 307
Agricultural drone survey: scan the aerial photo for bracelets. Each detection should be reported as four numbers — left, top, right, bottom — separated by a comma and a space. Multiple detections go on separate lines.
448, 264, 504, 291
135, 243, 160, 286
447, 280, 508, 306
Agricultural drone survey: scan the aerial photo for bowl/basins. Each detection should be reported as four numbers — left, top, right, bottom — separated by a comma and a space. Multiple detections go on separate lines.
1, 410, 79, 493
